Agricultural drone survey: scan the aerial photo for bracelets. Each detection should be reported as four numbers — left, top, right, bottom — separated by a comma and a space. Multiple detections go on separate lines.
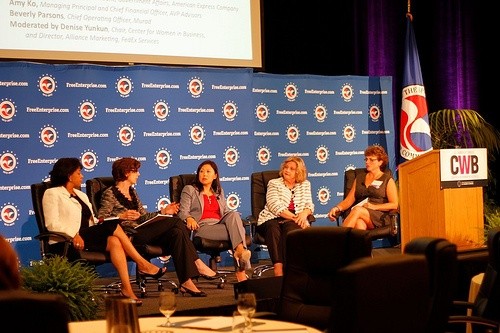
335, 205, 340, 213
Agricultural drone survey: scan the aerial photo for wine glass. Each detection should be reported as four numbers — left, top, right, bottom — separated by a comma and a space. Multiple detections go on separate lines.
158, 292, 177, 327
237, 293, 257, 333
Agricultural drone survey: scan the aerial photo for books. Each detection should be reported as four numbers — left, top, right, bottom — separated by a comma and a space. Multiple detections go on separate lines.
133, 213, 173, 231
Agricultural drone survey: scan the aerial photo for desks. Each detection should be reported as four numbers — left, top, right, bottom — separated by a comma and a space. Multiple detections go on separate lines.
68, 316, 326, 333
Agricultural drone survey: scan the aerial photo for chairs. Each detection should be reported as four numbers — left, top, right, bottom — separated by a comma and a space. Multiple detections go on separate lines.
0, 169, 500, 333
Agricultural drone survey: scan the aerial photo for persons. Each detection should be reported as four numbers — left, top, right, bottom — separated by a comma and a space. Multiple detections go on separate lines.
329, 146, 399, 229
180, 160, 252, 282
0, 235, 22, 293
259, 156, 315, 277
42, 157, 167, 306
98, 158, 225, 298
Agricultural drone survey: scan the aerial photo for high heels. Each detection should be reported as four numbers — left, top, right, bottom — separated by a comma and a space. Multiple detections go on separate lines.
235, 271, 247, 282
120, 291, 142, 306
138, 267, 167, 279
196, 271, 224, 282
179, 285, 207, 297
234, 249, 251, 267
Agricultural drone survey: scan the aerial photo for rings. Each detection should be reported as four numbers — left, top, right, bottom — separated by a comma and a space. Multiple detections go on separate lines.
76, 244, 79, 245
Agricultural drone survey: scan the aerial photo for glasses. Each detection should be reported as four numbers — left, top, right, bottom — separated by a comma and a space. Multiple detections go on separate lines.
364, 158, 378, 161
131, 169, 138, 173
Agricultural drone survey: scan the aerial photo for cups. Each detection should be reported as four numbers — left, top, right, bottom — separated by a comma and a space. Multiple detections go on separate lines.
104, 294, 141, 333
232, 311, 252, 333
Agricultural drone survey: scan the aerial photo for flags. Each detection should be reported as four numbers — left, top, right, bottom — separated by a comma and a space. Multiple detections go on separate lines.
399, 17, 433, 163
